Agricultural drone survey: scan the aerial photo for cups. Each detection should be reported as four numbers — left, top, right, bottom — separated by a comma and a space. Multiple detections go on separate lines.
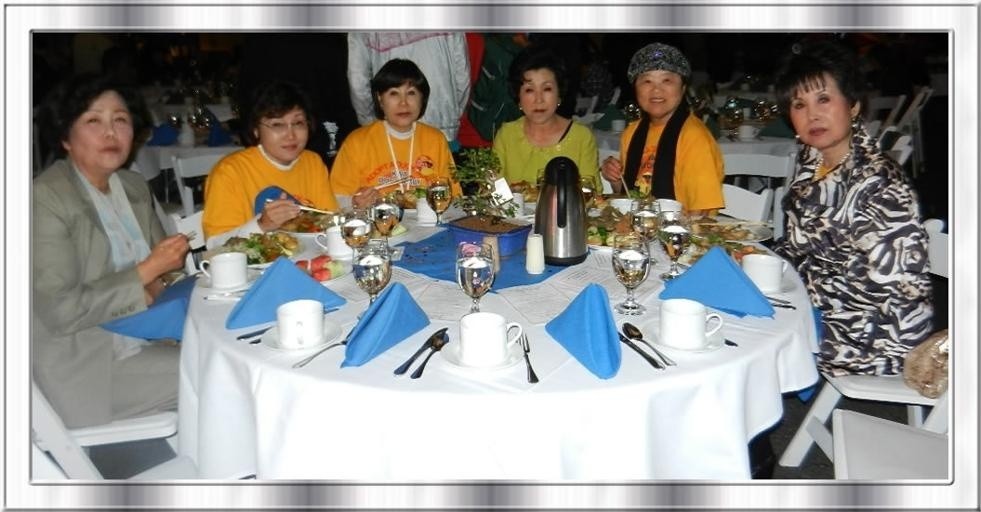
584, 176, 596, 210
201, 251, 249, 290
509, 193, 525, 217
613, 121, 625, 130
459, 313, 522, 366
738, 127, 758, 142
741, 255, 788, 296
316, 227, 358, 260
658, 300, 721, 350
277, 300, 325, 348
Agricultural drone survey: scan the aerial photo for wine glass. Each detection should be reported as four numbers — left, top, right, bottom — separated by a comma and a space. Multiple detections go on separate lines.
341, 207, 372, 265
455, 241, 495, 319
613, 232, 648, 314
427, 178, 452, 226
375, 195, 398, 256
631, 199, 660, 266
354, 246, 390, 323
658, 212, 689, 283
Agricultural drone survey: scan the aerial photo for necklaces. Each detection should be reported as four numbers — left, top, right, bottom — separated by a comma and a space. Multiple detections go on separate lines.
814, 151, 851, 180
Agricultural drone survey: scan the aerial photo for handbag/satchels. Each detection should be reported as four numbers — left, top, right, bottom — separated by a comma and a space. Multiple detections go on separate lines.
903, 327, 948, 396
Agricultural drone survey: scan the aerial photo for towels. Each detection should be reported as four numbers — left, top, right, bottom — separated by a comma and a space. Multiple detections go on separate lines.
99, 276, 198, 343
759, 117, 796, 139
592, 102, 628, 130
546, 283, 621, 379
145, 123, 179, 146
659, 247, 774, 318
226, 257, 345, 328
342, 285, 430, 369
209, 124, 232, 147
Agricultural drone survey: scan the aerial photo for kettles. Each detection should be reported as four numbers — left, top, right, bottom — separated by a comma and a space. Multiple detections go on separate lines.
535, 156, 588, 265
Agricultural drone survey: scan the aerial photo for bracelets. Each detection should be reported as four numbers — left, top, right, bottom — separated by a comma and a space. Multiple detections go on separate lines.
159, 277, 174, 285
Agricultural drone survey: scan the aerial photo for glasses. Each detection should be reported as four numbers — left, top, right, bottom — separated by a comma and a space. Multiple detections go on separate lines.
256, 116, 310, 134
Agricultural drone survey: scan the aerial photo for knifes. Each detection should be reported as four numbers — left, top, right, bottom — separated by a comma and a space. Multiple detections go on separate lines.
393, 327, 448, 376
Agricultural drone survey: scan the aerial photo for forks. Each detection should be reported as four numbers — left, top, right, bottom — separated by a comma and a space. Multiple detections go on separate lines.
516, 334, 538, 382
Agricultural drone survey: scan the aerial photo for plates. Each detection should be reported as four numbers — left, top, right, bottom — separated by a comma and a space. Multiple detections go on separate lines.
247, 232, 327, 268
688, 219, 772, 247
263, 322, 344, 348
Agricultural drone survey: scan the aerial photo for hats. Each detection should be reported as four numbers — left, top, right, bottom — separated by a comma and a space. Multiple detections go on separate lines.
627, 42, 691, 84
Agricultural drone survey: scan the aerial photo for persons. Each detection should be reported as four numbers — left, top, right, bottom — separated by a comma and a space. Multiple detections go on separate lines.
749, 59, 935, 480
328, 59, 463, 209
203, 87, 341, 252
31, 79, 191, 429
489, 51, 603, 200
602, 43, 727, 215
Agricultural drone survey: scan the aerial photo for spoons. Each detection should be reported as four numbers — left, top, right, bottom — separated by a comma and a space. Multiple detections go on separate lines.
411, 332, 450, 379
623, 322, 676, 366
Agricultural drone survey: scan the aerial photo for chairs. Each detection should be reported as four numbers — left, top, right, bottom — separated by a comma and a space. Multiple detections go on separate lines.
171, 210, 203, 274
172, 155, 224, 216
717, 184, 773, 228
866, 87, 934, 176
831, 380, 948, 482
31, 377, 178, 479
779, 221, 949, 466
